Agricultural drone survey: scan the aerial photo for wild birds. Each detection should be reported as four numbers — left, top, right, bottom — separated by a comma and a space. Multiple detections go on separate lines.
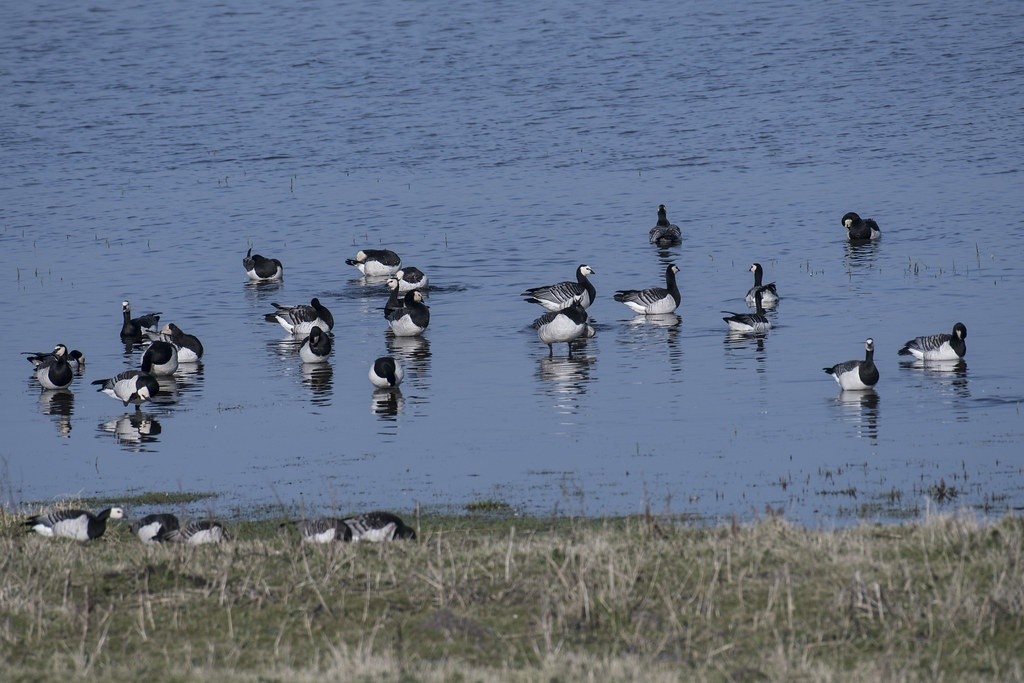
520, 264, 595, 325
720, 288, 773, 334
745, 262, 779, 311
897, 321, 968, 361
15, 505, 418, 548
822, 337, 879, 391
529, 292, 596, 360
20, 246, 432, 412
613, 264, 681, 315
841, 212, 881, 241
648, 204, 682, 250
